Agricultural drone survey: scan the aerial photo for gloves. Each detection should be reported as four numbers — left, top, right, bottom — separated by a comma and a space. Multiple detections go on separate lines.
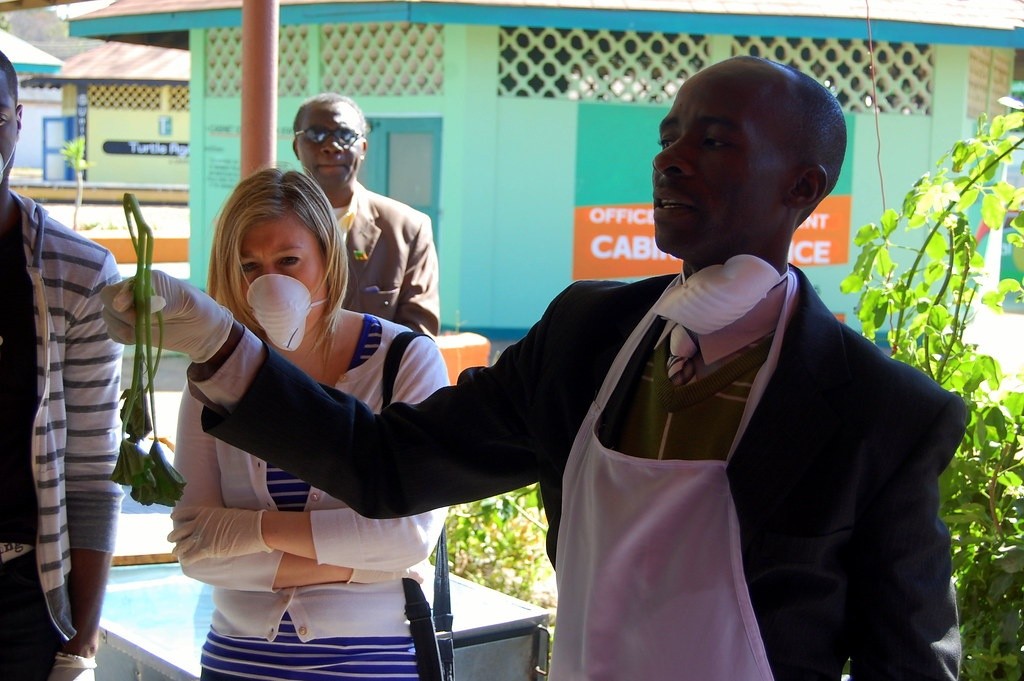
98, 268, 235, 364
347, 562, 424, 585
49, 651, 97, 681
167, 505, 275, 567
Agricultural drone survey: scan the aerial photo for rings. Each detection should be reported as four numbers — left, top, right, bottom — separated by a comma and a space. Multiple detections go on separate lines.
192, 532, 200, 542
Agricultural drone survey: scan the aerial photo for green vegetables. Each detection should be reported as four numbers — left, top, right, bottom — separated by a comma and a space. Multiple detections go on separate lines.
113, 192, 185, 506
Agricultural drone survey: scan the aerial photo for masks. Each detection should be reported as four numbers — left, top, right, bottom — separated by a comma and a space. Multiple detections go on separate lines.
658, 252, 790, 336
237, 273, 329, 352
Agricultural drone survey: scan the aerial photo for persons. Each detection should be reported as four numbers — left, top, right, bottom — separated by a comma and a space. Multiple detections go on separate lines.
100, 55, 972, 681
292, 93, 441, 341
167, 168, 451, 681
0, 51, 122, 681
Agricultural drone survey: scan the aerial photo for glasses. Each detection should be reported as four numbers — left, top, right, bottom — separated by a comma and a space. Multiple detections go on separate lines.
295, 125, 364, 146
